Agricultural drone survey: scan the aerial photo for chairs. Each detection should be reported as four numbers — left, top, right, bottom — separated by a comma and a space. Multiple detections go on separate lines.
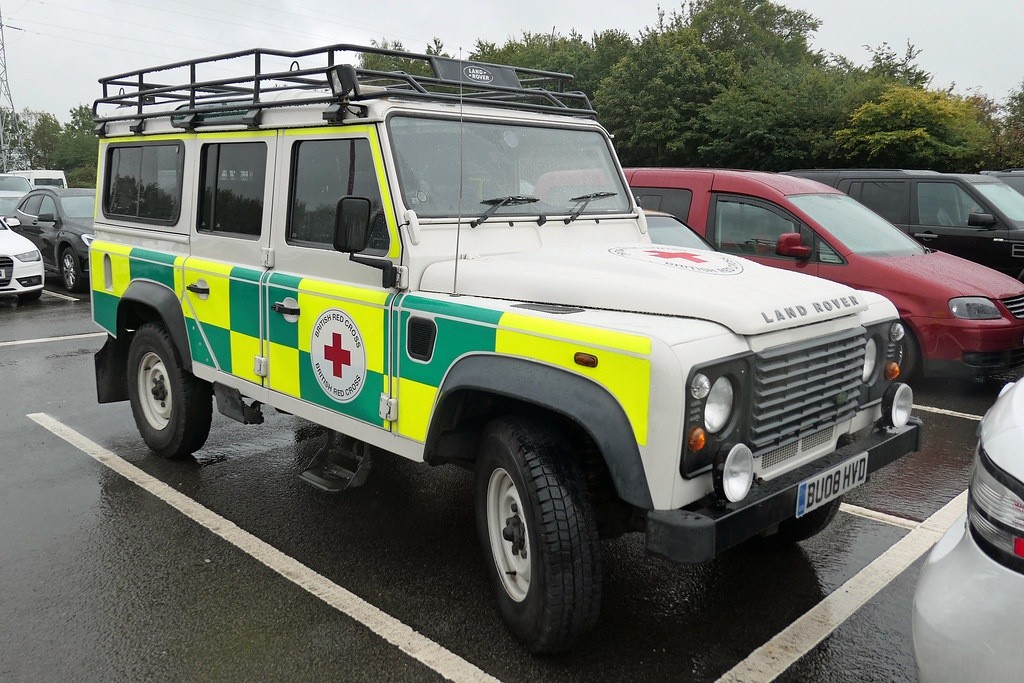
932, 196, 953, 226
748, 214, 778, 243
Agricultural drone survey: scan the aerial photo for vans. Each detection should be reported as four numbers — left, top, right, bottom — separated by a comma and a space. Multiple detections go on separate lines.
7, 170, 68, 189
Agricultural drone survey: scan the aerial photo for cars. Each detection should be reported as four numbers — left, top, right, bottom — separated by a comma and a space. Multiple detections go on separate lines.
979, 169, 1024, 197
910, 377, 1023, 682
532, 167, 1024, 386
2, 187, 97, 293
0, 190, 30, 220
782, 168, 1024, 281
0, 217, 44, 300
0, 174, 34, 193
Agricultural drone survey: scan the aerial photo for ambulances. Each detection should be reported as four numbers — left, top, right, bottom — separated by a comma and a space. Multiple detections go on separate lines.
87, 43, 926, 658
